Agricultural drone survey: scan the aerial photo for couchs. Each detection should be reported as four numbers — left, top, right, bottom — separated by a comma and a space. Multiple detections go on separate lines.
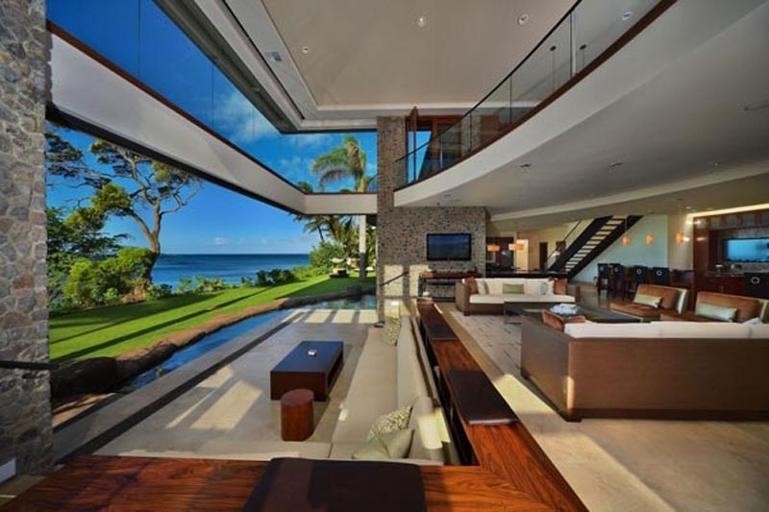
609, 283, 690, 323
519, 309, 769, 424
455, 278, 581, 316
660, 291, 769, 323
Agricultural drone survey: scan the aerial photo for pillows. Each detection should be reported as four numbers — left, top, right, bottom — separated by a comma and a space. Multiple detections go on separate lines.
465, 276, 487, 294
382, 316, 400, 345
350, 406, 414, 459
537, 275, 569, 296
502, 284, 524, 293
695, 302, 738, 322
632, 293, 662, 308
540, 310, 564, 333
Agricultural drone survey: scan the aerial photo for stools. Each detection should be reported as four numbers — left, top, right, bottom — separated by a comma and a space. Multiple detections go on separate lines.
280, 389, 315, 442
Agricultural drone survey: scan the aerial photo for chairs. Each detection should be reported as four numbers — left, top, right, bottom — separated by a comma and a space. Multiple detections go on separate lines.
596, 260, 668, 301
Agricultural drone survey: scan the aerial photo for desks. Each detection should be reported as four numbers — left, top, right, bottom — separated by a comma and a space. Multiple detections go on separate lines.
418, 273, 483, 302
